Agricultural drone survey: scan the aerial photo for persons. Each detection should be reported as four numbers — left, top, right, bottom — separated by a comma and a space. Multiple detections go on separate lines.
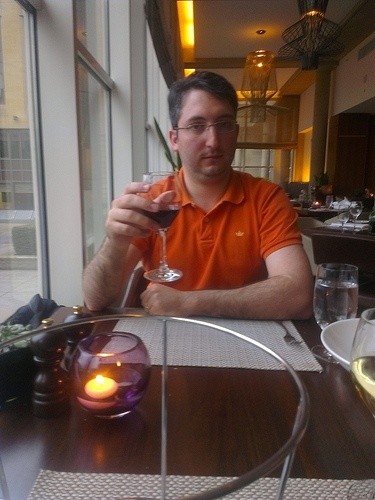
82, 70, 315, 320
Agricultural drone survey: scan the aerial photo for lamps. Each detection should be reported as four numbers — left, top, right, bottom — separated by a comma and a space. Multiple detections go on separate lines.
272, 0, 345, 70
237, 49, 288, 123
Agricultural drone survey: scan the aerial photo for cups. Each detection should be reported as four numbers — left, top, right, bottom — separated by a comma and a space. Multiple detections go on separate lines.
70, 330, 152, 419
350, 308, 375, 420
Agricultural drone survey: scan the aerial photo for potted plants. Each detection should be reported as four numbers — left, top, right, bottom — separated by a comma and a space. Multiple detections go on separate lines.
313, 168, 333, 194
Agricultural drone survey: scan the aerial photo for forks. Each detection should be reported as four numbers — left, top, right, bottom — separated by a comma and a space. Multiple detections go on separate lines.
275, 320, 303, 347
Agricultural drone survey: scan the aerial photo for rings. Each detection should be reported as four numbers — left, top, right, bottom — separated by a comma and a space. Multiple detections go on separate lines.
144, 307, 151, 310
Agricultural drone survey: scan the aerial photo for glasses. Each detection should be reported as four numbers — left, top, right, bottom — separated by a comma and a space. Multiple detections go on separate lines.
173, 119, 238, 137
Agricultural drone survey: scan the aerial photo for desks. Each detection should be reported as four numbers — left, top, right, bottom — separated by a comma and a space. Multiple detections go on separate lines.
290, 199, 375, 274
0, 307, 375, 500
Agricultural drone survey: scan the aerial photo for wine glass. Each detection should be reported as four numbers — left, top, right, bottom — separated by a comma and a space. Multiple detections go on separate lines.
141, 170, 184, 283
338, 205, 350, 234
349, 201, 363, 232
313, 262, 359, 363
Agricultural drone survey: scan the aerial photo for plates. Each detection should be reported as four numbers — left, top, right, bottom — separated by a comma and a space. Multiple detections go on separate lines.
320, 316, 375, 372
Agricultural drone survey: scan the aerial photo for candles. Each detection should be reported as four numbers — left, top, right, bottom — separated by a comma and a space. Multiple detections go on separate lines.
84, 373, 118, 400
